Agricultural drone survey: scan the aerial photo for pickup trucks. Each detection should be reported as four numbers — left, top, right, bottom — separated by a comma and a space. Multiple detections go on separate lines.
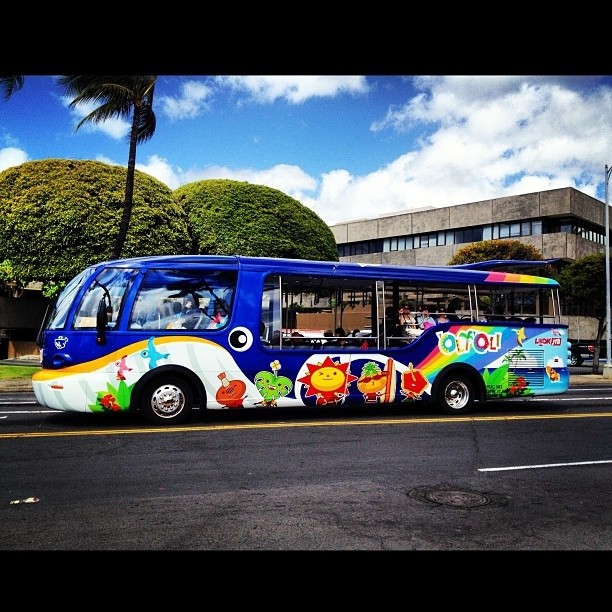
568, 342, 594, 367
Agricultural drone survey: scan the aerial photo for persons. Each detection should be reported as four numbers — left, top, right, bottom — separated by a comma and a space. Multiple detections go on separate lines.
436, 307, 450, 324
208, 299, 229, 329
417, 305, 435, 329
335, 327, 346, 346
398, 305, 416, 329
182, 301, 203, 329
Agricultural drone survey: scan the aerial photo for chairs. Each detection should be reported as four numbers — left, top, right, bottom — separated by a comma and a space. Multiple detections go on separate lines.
263, 339, 401, 350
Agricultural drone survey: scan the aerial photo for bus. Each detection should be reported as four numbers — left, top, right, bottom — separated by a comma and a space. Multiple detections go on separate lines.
29, 255, 576, 425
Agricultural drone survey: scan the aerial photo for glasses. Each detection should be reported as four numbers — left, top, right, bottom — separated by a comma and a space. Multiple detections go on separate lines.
403, 307, 410, 310
439, 309, 445, 313
